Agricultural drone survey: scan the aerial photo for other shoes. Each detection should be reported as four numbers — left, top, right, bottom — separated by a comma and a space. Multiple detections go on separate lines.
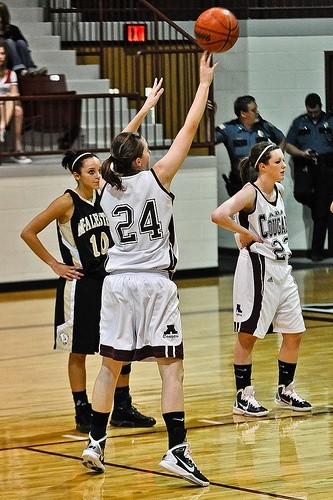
26, 67, 48, 75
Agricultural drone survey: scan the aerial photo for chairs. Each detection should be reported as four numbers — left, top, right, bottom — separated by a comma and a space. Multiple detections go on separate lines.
18, 75, 82, 148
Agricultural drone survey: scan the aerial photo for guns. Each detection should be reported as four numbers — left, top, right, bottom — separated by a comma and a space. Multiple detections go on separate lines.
222, 173, 234, 197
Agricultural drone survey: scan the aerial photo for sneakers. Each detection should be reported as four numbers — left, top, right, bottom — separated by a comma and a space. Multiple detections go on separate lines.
159, 441, 210, 487
110, 405, 156, 427
10, 150, 33, 164
274, 381, 313, 412
233, 386, 269, 417
81, 432, 107, 473
75, 403, 92, 433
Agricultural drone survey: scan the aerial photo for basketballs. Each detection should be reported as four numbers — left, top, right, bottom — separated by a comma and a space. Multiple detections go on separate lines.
193, 6, 242, 55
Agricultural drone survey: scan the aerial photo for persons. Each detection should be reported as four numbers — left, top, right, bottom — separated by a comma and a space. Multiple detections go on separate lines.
210, 143, 314, 418
0, 0, 219, 487
204, 96, 287, 197
285, 93, 333, 261
